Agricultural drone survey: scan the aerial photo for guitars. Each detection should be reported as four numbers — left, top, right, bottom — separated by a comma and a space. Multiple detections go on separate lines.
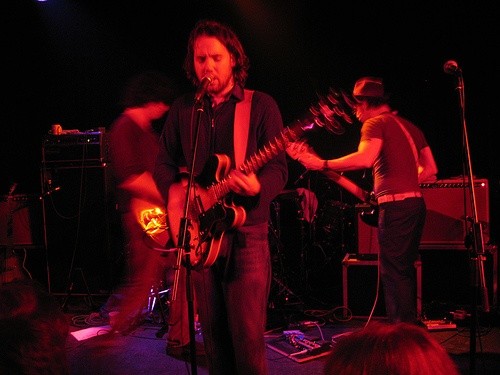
129, 86, 360, 271
285, 139, 379, 227
0, 183, 32, 283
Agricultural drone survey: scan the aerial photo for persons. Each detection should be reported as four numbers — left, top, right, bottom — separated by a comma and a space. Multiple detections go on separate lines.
298, 79, 438, 331
316, 323, 460, 375
92, 21, 289, 375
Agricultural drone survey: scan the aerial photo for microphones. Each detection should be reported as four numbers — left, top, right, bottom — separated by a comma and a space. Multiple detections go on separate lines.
294, 169, 310, 185
194, 73, 214, 104
443, 60, 462, 74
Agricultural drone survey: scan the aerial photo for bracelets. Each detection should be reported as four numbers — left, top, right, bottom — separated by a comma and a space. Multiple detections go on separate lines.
323, 158, 329, 171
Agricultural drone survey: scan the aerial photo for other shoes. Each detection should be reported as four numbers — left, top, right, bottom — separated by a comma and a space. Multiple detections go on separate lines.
167, 341, 206, 366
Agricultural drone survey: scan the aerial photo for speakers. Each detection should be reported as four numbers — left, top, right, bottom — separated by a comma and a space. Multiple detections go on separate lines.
38, 163, 127, 300
418, 180, 489, 245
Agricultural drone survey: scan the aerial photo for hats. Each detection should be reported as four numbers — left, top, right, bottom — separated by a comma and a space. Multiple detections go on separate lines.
351, 78, 384, 98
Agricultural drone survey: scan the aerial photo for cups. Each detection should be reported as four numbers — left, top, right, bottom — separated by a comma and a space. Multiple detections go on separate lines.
52, 125, 61, 135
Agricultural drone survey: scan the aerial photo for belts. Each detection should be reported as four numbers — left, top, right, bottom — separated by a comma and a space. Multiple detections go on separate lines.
377, 191, 423, 204
